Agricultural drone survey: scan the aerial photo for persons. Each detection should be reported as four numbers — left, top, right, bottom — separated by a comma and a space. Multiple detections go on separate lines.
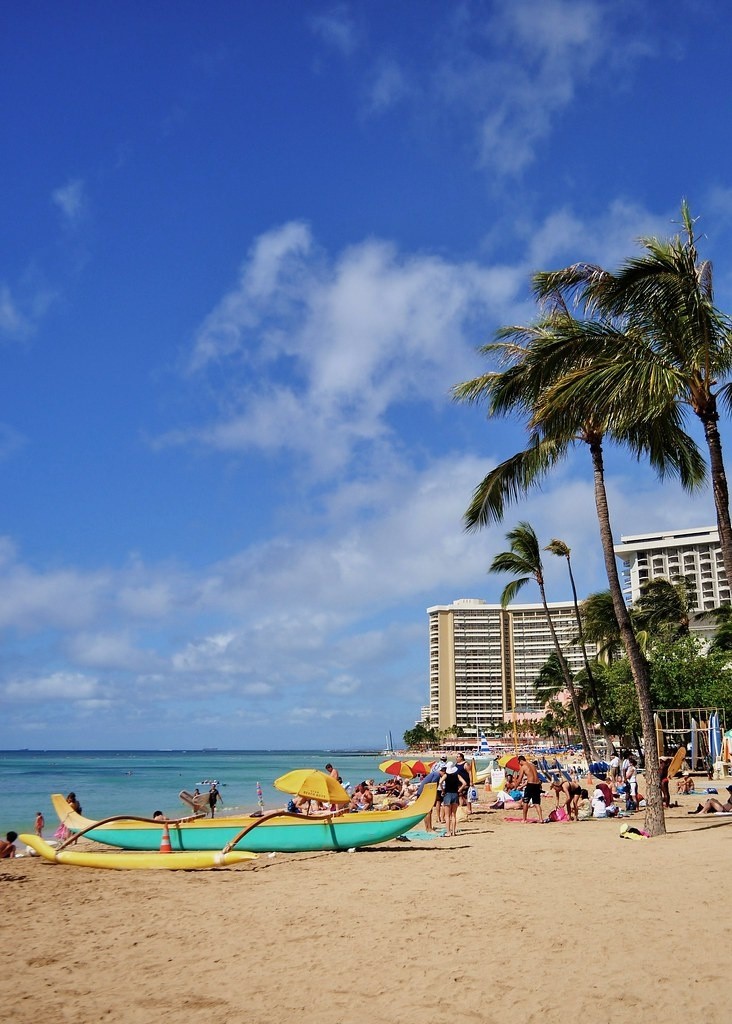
430, 753, 448, 824
192, 789, 201, 816
34, 812, 46, 842
0, 831, 18, 860
437, 760, 466, 837
675, 771, 694, 795
474, 745, 674, 823
453, 752, 475, 816
151, 810, 169, 821
285, 793, 311, 814
418, 767, 446, 834
308, 764, 428, 815
208, 784, 223, 819
54, 793, 82, 846
697, 784, 732, 814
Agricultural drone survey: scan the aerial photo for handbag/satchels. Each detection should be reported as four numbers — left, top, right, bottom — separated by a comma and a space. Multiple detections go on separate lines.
548, 807, 569, 822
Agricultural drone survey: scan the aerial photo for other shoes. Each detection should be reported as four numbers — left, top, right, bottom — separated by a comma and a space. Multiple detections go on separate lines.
396, 835, 411, 842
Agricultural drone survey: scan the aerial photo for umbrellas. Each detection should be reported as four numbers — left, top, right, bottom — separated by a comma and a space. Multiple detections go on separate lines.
497, 754, 521, 772
256, 782, 265, 815
379, 759, 438, 782
470, 758, 478, 784
276, 768, 350, 817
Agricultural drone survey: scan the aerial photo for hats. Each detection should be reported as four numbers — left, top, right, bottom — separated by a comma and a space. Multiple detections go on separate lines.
440, 754, 447, 759
726, 784, 732, 792
445, 761, 458, 774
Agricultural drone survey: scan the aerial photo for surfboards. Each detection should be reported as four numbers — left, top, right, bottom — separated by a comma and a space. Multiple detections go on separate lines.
178, 789, 210, 814
192, 792, 218, 805
689, 710, 721, 770
655, 715, 664, 767
534, 756, 608, 781
667, 747, 686, 778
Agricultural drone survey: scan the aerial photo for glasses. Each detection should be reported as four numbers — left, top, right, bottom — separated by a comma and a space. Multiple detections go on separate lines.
518, 760, 520, 764
456, 756, 460, 758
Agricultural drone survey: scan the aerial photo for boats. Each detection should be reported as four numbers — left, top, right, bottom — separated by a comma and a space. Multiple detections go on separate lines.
18, 833, 260, 870
52, 783, 436, 851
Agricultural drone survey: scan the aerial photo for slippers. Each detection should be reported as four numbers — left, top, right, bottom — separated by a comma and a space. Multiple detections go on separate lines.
451, 832, 456, 836
440, 833, 450, 837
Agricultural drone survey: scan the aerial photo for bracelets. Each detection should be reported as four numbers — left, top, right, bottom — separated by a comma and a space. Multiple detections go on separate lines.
521, 786, 524, 787
